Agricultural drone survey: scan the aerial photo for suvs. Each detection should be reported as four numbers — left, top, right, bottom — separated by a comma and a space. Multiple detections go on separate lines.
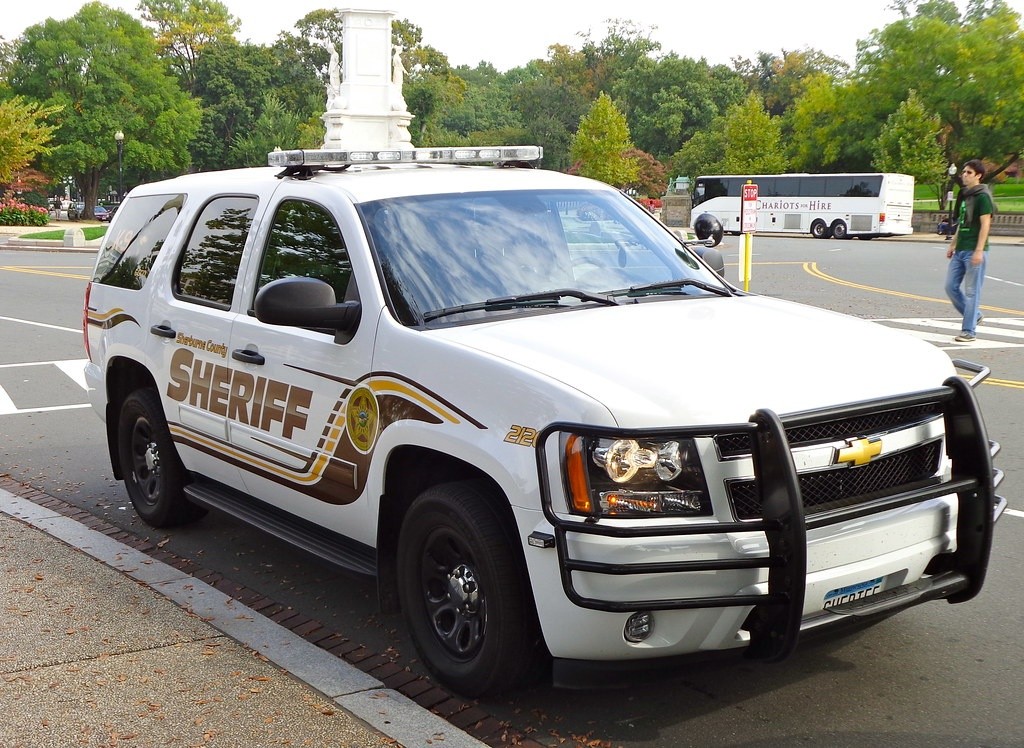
80, 141, 1010, 702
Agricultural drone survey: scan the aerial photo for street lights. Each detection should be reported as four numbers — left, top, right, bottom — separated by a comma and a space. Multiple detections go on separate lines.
944, 162, 957, 241
114, 130, 125, 205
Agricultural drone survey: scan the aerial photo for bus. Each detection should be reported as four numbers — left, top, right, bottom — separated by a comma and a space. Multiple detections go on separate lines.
688, 172, 915, 240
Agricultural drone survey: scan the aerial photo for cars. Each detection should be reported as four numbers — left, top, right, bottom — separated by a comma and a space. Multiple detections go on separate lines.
48, 196, 119, 222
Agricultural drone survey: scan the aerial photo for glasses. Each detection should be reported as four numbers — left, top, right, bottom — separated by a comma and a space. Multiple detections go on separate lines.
960, 170, 972, 175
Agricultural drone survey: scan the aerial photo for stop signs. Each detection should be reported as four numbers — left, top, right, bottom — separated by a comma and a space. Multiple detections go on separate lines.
739, 183, 759, 233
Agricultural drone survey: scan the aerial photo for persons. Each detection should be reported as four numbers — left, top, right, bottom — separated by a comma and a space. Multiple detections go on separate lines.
939, 215, 958, 235
54, 197, 62, 221
945, 160, 994, 342
648, 200, 655, 215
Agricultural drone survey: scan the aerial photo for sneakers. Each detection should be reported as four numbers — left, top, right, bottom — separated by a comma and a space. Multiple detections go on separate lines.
976, 312, 984, 325
955, 331, 976, 342
